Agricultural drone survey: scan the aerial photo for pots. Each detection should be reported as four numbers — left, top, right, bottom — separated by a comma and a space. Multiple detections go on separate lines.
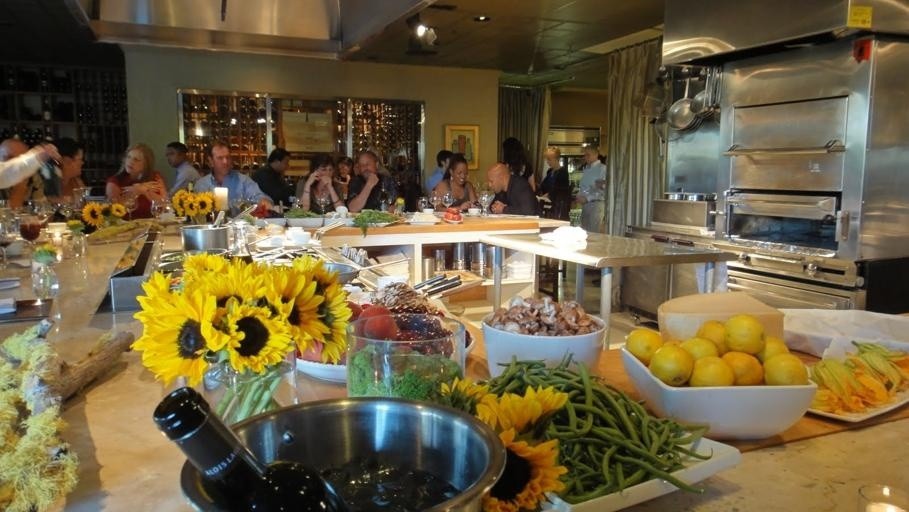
304, 257, 414, 282
179, 207, 229, 251
665, 75, 716, 132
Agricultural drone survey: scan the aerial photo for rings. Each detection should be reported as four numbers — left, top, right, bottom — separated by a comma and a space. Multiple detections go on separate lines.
152, 186, 154, 188
127, 190, 129, 192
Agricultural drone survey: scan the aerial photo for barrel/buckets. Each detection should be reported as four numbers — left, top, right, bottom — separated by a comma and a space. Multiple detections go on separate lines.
183, 224, 228, 252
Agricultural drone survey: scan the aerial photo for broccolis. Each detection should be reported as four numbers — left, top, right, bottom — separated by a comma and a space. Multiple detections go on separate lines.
352, 342, 463, 403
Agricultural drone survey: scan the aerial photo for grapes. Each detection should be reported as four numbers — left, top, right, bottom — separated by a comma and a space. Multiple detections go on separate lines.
381, 305, 453, 358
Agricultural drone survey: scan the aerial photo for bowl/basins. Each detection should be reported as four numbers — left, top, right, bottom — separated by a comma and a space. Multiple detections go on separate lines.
656, 290, 786, 339
483, 311, 605, 387
265, 225, 311, 245
619, 339, 818, 441
158, 247, 227, 272
253, 215, 358, 228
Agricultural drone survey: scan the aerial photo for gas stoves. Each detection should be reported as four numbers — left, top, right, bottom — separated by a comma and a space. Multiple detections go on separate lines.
247, 246, 392, 299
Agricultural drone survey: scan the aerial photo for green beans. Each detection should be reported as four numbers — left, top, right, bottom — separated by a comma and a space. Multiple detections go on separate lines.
478, 350, 713, 503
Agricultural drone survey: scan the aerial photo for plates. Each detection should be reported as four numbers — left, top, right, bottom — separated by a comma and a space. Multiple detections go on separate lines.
292, 331, 477, 383
1, 297, 54, 324
543, 412, 742, 510
779, 307, 908, 359
807, 391, 908, 424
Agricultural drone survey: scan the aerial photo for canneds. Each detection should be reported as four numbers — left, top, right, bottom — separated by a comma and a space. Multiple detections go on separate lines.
420, 197, 429, 212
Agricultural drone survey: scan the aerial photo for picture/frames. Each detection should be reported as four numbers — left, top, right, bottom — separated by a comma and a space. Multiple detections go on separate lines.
443, 123, 481, 171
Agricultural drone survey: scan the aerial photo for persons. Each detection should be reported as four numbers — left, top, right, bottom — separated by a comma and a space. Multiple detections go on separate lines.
251, 147, 296, 211
0, 138, 64, 194
425, 150, 456, 193
345, 150, 401, 213
0, 129, 46, 207
193, 138, 274, 217
165, 140, 203, 202
430, 152, 483, 213
332, 155, 352, 199
104, 141, 168, 221
574, 144, 605, 234
486, 164, 543, 217
499, 137, 536, 198
293, 153, 347, 213
591, 177, 606, 287
537, 146, 570, 276
45, 136, 91, 206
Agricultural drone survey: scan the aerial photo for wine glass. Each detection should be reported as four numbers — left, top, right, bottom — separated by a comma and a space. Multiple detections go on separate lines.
233, 186, 496, 222
1, 187, 168, 308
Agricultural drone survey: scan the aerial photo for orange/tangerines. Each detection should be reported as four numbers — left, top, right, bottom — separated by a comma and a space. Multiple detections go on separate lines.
624, 314, 808, 388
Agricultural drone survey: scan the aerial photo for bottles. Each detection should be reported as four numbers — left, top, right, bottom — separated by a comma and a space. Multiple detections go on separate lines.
342, 243, 367, 266
228, 222, 252, 259
152, 386, 345, 512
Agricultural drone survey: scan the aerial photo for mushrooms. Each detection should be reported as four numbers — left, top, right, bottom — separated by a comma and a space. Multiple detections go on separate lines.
483, 292, 603, 337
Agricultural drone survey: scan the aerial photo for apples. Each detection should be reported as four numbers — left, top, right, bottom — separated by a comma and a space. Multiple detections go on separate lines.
444, 206, 463, 222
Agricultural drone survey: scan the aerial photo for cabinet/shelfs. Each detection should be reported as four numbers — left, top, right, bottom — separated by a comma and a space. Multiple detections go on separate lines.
1, 59, 128, 196
177, 89, 425, 200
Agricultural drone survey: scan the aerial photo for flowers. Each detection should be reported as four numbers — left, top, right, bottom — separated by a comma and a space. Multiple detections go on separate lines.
82, 201, 127, 227
172, 190, 227, 217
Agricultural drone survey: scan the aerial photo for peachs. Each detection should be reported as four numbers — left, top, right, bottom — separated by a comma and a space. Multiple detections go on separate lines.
302, 303, 398, 364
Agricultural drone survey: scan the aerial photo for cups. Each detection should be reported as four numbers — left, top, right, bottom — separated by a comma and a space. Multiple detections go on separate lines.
343, 315, 465, 396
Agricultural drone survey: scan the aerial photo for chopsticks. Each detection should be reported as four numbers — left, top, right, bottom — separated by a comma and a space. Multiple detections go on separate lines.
401, 270, 466, 301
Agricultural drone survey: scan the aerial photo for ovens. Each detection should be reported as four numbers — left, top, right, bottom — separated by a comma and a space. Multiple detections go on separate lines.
730, 92, 869, 322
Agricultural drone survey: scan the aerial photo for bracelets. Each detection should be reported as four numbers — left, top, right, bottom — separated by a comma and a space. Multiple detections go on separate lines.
303, 189, 310, 194
333, 199, 340, 204
